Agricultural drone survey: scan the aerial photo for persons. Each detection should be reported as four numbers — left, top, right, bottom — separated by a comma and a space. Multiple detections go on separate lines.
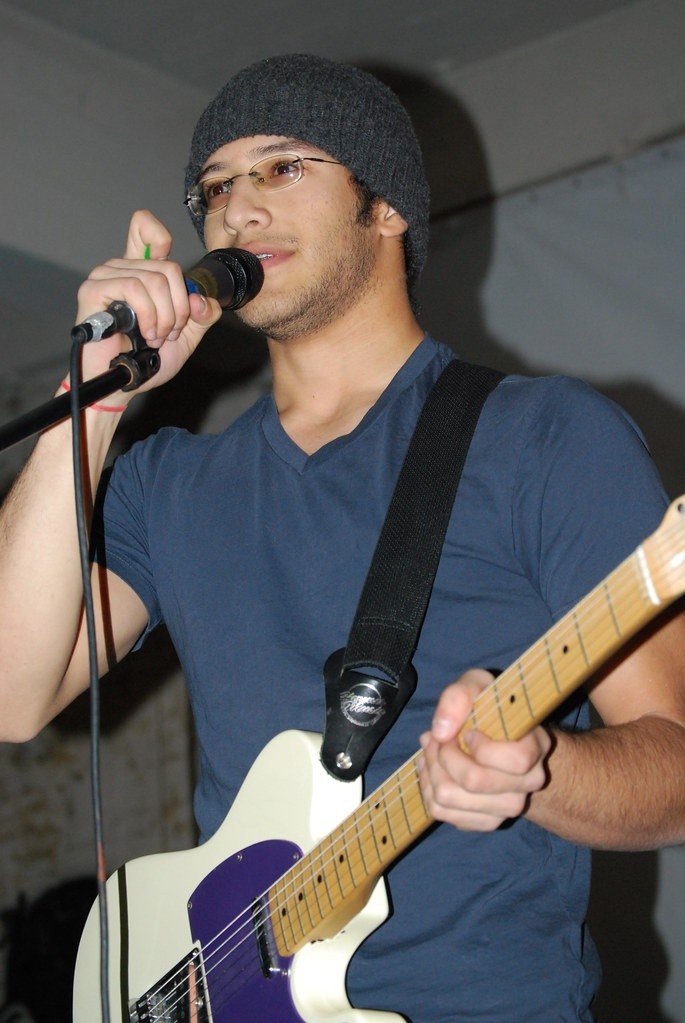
1, 54, 685, 1023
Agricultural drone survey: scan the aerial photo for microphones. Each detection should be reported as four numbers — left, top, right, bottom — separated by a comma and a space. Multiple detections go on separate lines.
72, 248, 265, 343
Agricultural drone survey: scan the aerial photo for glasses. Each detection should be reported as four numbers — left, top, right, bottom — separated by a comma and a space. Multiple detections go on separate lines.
183, 153, 345, 217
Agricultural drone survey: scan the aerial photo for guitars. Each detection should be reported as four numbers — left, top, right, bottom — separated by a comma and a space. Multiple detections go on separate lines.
70, 492, 685, 1023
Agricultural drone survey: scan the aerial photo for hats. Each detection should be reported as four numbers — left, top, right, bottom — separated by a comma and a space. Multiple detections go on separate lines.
184, 53, 432, 290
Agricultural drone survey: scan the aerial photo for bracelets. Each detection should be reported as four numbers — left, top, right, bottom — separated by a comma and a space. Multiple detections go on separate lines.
59, 381, 128, 413
